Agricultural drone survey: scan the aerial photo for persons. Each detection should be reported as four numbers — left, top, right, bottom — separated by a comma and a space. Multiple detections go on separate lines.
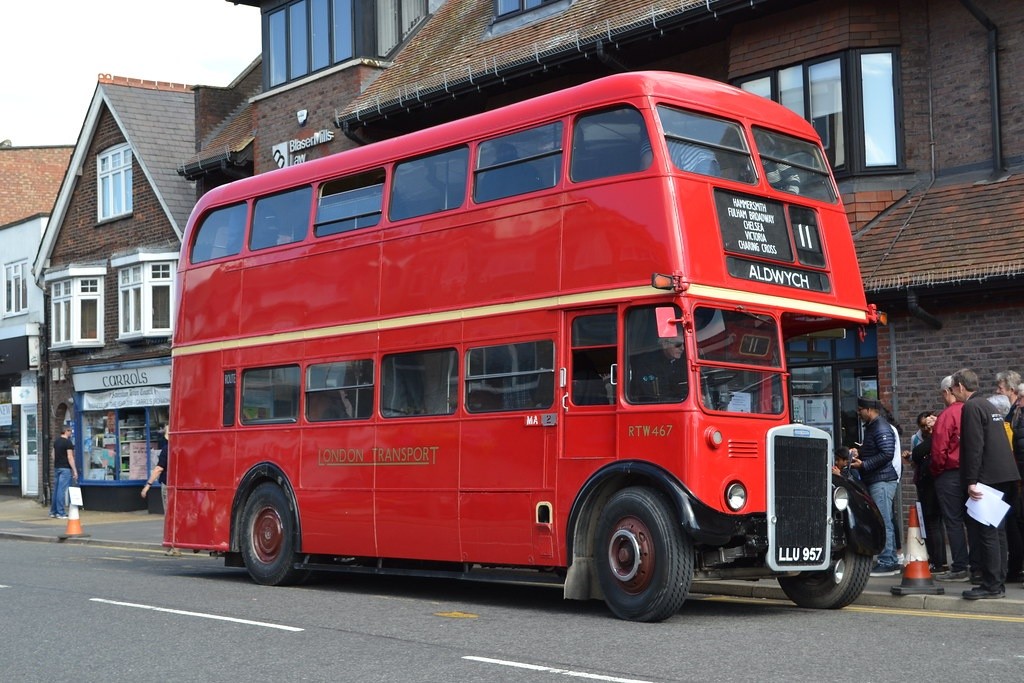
740, 149, 800, 196
949, 368, 1024, 601
931, 375, 970, 582
141, 425, 183, 557
637, 118, 720, 177
832, 396, 938, 577
632, 330, 707, 403
47, 425, 78, 519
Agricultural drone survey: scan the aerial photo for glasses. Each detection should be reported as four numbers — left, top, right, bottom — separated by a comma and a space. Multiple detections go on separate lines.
858, 407, 864, 412
948, 383, 957, 392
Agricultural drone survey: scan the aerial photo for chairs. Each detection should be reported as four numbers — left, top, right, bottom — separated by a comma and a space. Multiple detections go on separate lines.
226, 125, 593, 259
227, 353, 610, 426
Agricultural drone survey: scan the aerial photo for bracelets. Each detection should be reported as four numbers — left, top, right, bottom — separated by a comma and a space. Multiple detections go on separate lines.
147, 482, 152, 485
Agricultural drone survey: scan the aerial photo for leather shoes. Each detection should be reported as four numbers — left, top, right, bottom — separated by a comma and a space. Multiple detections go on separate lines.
962, 588, 1001, 599
972, 587, 1005, 598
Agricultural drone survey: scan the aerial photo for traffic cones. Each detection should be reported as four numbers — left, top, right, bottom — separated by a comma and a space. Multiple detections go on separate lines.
57, 499, 92, 539
889, 506, 947, 596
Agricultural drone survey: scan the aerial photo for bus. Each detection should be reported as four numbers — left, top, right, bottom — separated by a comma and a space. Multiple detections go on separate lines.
159, 67, 888, 626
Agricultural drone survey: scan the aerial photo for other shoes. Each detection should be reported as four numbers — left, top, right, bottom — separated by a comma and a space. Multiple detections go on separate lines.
50, 514, 56, 518
929, 565, 950, 574
165, 548, 181, 556
936, 571, 970, 582
56, 513, 68, 519
895, 564, 900, 575
869, 564, 895, 577
1004, 570, 1024, 584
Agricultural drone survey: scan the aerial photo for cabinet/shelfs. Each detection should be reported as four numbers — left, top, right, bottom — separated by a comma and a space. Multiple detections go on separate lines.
119, 424, 164, 474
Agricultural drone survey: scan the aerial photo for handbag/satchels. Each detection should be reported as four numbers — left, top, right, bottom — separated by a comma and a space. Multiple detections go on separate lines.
65, 481, 83, 507
